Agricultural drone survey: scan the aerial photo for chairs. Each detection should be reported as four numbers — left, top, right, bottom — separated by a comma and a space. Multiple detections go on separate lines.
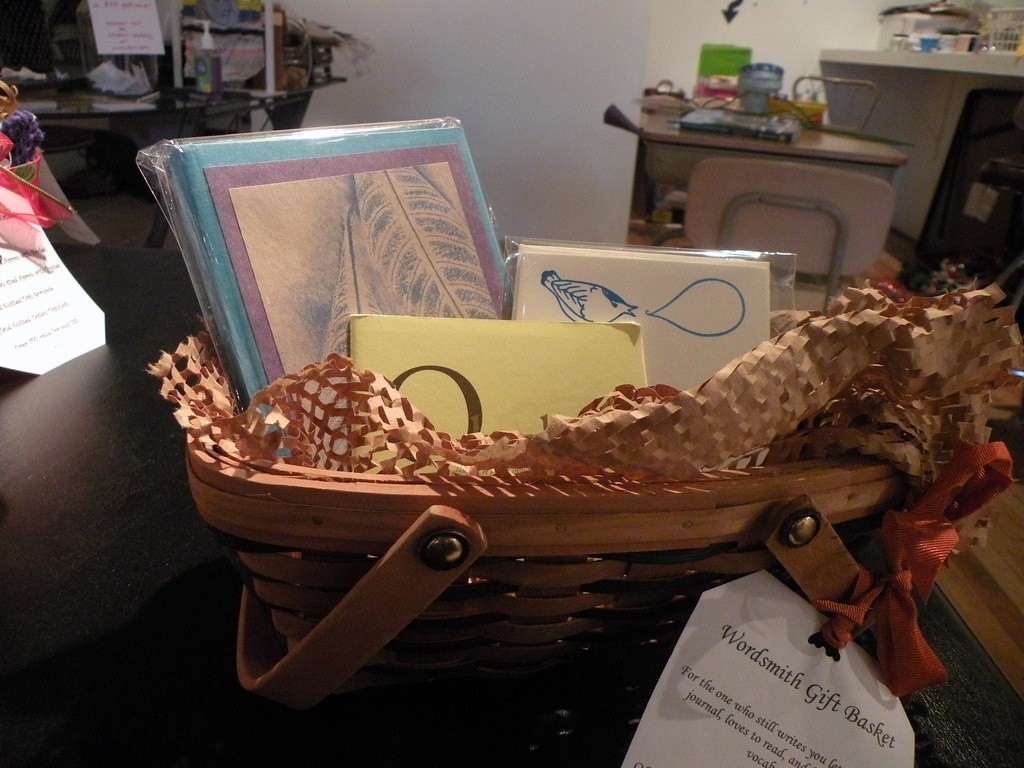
650, 156, 898, 315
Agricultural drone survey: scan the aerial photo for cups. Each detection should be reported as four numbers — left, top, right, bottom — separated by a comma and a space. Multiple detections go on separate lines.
738, 62, 784, 112
891, 33, 974, 53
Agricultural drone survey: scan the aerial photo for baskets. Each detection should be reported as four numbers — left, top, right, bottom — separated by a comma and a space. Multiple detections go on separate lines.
185, 392, 960, 768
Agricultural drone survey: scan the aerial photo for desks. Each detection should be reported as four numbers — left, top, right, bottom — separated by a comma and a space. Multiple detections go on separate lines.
0, 242, 1024, 768
630, 95, 911, 289
11, 72, 349, 249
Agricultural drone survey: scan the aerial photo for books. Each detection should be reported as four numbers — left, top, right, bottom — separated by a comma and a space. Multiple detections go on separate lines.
152, 116, 771, 460
681, 108, 800, 142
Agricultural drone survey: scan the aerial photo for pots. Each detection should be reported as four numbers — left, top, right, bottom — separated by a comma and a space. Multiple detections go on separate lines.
880, 0, 984, 54
